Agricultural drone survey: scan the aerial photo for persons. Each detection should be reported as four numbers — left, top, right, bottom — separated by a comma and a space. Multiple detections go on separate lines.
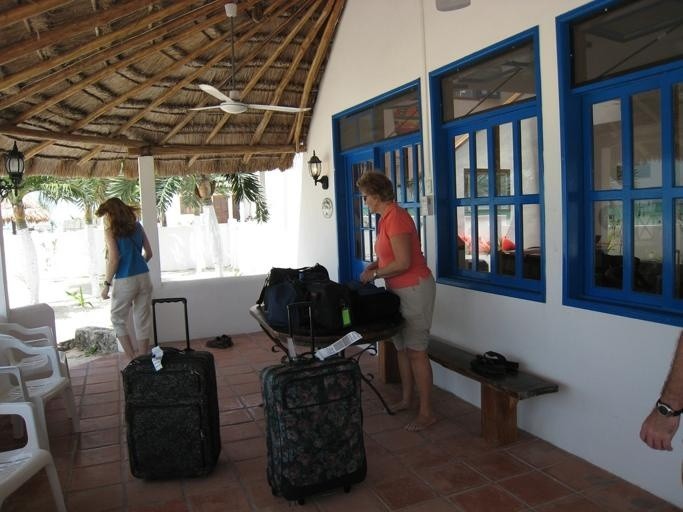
356, 171, 438, 432
95, 196, 152, 373
639, 329, 683, 487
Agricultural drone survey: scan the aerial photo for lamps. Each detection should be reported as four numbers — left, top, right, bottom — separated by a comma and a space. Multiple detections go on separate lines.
0, 141, 26, 198
307, 149, 328, 191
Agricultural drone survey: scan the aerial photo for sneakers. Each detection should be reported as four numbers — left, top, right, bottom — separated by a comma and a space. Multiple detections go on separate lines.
206, 333, 233, 350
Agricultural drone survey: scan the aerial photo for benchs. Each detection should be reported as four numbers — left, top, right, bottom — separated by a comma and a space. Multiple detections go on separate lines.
373, 335, 561, 447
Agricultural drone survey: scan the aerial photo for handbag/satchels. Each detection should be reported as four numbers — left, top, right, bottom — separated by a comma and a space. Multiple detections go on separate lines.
264, 263, 406, 336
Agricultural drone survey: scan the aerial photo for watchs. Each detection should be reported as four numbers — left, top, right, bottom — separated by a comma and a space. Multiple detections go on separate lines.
103, 280, 111, 287
372, 269, 379, 279
654, 399, 683, 416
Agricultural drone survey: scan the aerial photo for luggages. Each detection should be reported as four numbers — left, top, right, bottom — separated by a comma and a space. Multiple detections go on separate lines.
260, 301, 367, 506
120, 297, 220, 478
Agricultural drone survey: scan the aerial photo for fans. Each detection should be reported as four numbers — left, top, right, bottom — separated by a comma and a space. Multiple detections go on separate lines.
184, 4, 312, 116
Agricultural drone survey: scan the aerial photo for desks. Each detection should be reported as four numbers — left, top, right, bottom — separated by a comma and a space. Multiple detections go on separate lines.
251, 300, 398, 420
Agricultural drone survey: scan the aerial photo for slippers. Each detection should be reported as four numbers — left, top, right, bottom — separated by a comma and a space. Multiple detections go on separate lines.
471, 350, 519, 381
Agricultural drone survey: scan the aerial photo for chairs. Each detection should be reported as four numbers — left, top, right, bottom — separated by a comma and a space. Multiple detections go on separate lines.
0, 302, 81, 512
